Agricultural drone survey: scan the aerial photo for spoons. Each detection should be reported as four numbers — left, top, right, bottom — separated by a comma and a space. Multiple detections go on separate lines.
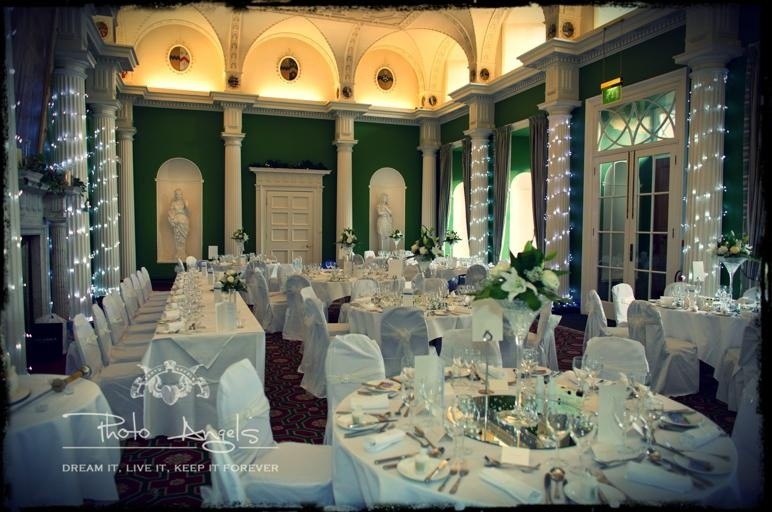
549, 466, 565, 498
343, 418, 397, 438
423, 456, 472, 496
588, 465, 631, 500
646, 439, 713, 489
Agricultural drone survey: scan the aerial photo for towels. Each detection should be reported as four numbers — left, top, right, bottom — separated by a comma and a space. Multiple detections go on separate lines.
623, 459, 697, 495
673, 423, 721, 451
482, 464, 542, 505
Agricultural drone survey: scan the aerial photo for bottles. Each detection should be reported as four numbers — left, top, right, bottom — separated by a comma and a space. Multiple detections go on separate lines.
291, 255, 303, 266
396, 346, 664, 472
647, 277, 760, 316
351, 279, 472, 316
201, 260, 223, 305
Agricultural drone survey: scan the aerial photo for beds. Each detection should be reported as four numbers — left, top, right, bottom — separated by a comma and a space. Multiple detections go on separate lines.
327, 369, 742, 508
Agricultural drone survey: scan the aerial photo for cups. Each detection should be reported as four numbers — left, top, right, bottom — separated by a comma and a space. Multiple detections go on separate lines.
154, 261, 206, 335
432, 254, 484, 274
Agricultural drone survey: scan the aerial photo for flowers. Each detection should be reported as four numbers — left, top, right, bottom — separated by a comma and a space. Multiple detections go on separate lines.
707, 229, 762, 265
230, 229, 249, 242
388, 229, 405, 242
465, 237, 578, 314
403, 225, 463, 260
336, 228, 360, 249
442, 227, 461, 246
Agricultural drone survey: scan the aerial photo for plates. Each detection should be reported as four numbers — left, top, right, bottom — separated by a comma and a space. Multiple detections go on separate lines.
673, 450, 734, 475
563, 479, 625, 507
11, 390, 31, 405
367, 379, 401, 393
661, 408, 706, 428
334, 412, 379, 432
398, 457, 450, 481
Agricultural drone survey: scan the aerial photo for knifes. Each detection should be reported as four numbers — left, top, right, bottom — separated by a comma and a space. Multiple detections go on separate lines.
596, 484, 610, 506
543, 471, 551, 504
374, 453, 419, 465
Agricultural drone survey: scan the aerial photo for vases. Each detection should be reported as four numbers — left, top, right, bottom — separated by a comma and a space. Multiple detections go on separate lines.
394, 238, 401, 245
718, 257, 748, 297
342, 243, 356, 255
237, 242, 243, 250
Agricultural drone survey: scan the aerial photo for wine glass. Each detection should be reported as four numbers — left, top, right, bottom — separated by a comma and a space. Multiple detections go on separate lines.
300, 261, 388, 280
211, 253, 279, 267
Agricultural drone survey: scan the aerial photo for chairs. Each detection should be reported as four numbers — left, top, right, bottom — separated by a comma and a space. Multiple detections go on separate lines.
70, 250, 564, 440
577, 281, 764, 415
321, 330, 386, 446
731, 380, 758, 487
216, 355, 336, 511
201, 424, 257, 509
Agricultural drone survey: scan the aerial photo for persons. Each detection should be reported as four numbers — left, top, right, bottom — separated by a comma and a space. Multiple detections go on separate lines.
375, 193, 392, 251
168, 189, 191, 259
